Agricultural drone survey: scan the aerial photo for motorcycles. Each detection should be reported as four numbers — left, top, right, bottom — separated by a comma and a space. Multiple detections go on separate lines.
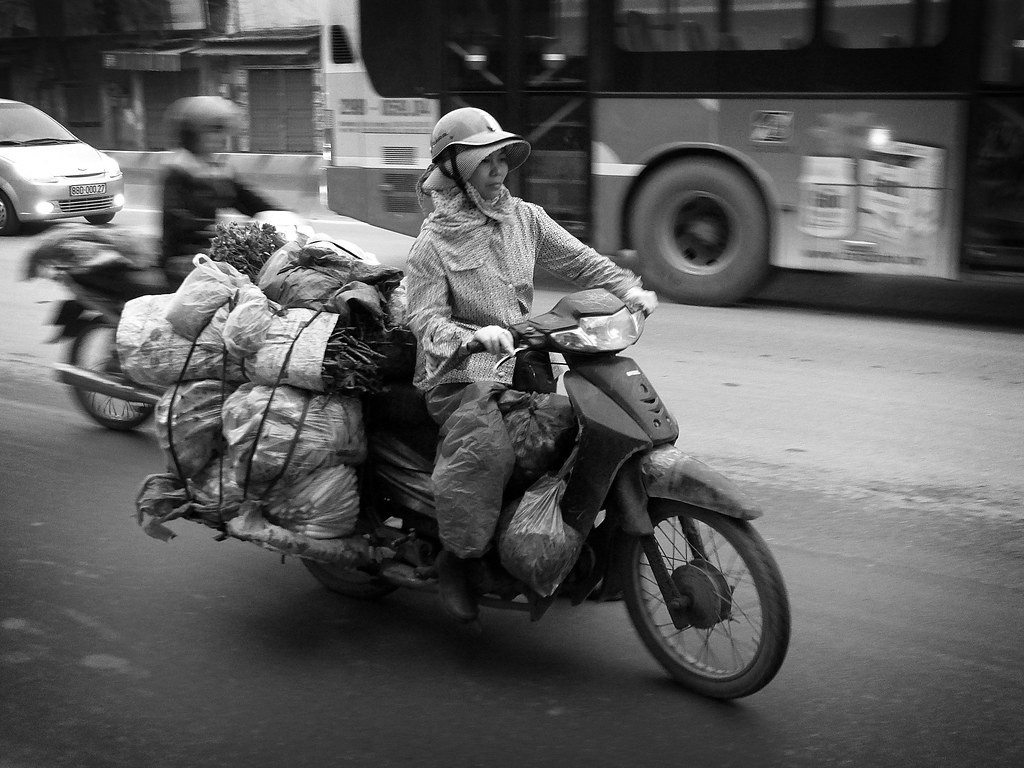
299, 287, 791, 698
34, 210, 306, 437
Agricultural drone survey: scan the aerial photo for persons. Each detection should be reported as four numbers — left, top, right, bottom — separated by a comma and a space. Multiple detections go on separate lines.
161, 96, 294, 291
407, 107, 658, 622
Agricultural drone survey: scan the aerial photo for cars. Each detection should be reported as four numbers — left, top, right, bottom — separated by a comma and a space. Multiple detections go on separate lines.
0, 98, 125, 237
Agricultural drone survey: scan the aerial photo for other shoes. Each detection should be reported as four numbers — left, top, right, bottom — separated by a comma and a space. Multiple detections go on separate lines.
435, 546, 484, 619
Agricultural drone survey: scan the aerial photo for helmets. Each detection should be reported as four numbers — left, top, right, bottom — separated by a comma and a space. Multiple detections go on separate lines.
427, 107, 518, 162
178, 95, 238, 148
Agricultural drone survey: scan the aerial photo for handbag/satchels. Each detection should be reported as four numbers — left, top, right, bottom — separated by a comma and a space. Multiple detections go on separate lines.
499, 388, 579, 477
498, 472, 582, 597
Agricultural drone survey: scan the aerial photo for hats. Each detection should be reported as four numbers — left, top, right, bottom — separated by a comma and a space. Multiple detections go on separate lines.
420, 138, 532, 193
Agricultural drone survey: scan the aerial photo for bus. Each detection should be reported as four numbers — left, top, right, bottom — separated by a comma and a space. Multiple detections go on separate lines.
323, 0, 1023, 330
